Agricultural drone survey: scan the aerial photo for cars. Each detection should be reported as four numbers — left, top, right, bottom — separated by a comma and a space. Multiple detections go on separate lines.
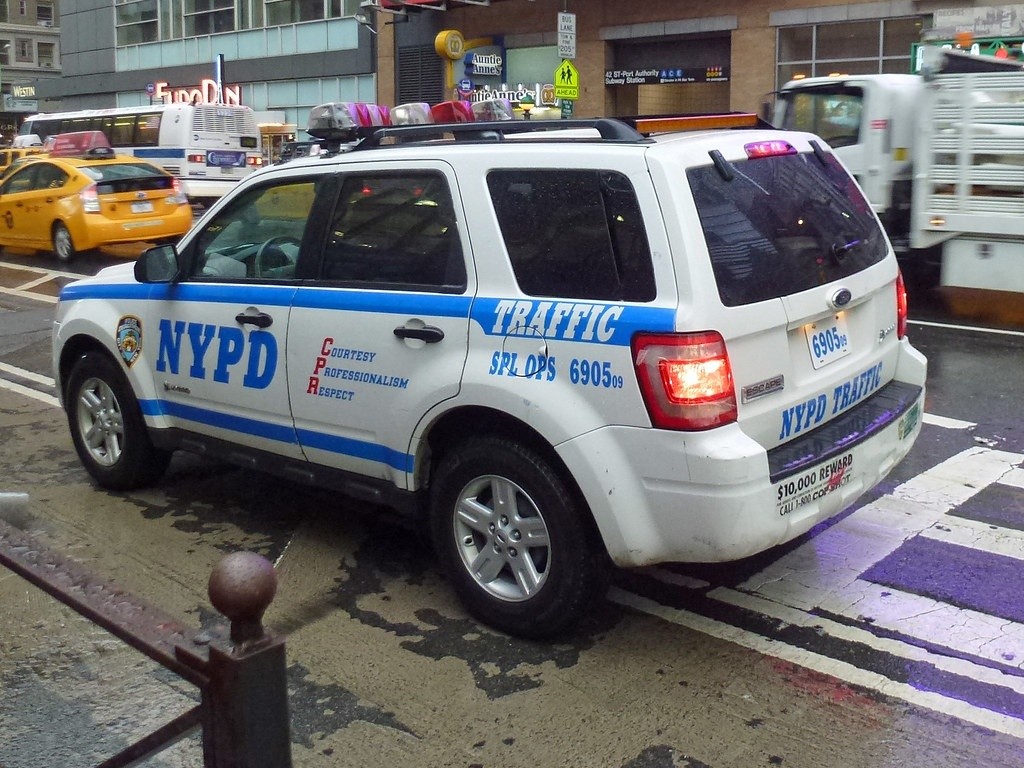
0, 134, 47, 181
238, 179, 324, 231
0, 131, 195, 263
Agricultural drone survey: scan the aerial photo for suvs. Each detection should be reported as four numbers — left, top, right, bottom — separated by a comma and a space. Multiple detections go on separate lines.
49, 96, 928, 645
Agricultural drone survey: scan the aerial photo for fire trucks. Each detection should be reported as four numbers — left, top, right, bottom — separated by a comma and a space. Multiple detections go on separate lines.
753, 7, 1024, 337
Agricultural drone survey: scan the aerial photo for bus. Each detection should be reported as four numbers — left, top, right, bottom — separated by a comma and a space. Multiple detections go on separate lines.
257, 122, 297, 167
11, 101, 260, 209
